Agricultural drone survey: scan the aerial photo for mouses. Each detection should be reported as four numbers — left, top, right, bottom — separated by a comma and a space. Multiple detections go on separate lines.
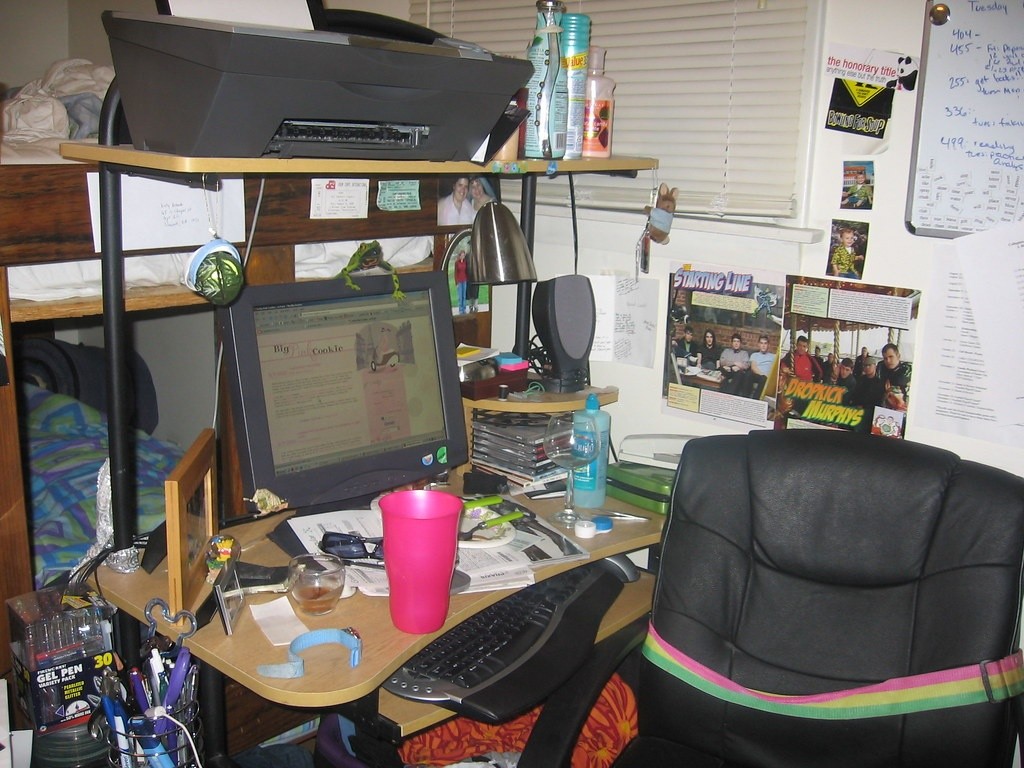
592, 553, 642, 584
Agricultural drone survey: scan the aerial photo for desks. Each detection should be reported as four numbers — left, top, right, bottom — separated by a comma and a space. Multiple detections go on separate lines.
59, 141, 659, 553
87, 468, 667, 768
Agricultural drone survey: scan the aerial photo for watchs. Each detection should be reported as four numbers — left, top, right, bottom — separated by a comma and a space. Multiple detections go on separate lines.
257, 626, 363, 679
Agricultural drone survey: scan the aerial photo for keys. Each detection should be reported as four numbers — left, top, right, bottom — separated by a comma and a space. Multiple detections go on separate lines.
636, 249, 640, 283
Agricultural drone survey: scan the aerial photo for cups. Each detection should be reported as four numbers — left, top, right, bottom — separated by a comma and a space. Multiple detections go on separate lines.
677, 358, 687, 375
378, 489, 463, 634
491, 101, 519, 160
289, 553, 345, 616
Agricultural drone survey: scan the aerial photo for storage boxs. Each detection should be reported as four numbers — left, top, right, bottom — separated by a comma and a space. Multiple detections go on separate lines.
4, 582, 117, 739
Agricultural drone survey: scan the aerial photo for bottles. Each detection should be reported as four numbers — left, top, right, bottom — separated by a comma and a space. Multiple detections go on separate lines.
516, 0, 569, 158
572, 394, 611, 509
562, 14, 590, 160
582, 46, 615, 158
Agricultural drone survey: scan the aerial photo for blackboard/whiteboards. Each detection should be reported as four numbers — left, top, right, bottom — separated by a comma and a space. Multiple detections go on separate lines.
906, 1, 1023, 238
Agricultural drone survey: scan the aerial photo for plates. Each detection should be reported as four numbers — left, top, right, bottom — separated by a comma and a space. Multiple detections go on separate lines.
370, 491, 516, 548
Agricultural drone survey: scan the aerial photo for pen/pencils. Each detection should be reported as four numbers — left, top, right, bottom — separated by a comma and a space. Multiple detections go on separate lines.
99, 646, 201, 768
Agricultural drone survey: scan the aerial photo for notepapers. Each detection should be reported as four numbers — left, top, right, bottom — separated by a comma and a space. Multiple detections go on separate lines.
495, 352, 529, 371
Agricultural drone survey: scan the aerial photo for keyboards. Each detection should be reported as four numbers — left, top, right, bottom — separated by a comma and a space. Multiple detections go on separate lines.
381, 565, 625, 721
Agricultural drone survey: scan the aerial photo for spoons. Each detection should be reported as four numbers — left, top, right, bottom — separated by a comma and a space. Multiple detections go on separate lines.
457, 511, 523, 541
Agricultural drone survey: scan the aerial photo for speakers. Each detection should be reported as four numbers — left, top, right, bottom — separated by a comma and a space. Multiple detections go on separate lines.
532, 275, 596, 394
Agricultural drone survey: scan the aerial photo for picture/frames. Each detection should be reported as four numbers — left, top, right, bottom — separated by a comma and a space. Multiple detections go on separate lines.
164, 426, 219, 628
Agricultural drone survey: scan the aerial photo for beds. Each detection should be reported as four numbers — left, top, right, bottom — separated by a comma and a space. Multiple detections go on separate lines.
0, 79, 502, 756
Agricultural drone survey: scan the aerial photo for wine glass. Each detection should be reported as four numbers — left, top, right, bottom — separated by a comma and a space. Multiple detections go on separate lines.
543, 413, 601, 529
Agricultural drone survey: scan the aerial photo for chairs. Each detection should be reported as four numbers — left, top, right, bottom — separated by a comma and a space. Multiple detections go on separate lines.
516, 430, 1024, 768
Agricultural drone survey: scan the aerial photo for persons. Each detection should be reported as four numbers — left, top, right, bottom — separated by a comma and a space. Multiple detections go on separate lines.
454, 240, 479, 315
776, 335, 912, 440
437, 174, 498, 225
841, 172, 873, 208
671, 325, 777, 400
830, 227, 865, 280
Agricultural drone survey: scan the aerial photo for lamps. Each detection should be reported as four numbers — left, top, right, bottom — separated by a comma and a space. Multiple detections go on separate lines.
440, 201, 538, 286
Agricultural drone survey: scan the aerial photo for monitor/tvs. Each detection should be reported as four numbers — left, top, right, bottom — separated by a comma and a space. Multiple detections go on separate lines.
215, 270, 469, 513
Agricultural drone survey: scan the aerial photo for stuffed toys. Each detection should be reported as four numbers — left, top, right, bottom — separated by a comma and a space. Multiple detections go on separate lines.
644, 183, 679, 246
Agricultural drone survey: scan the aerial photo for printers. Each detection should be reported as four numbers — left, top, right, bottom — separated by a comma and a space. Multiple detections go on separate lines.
101, 0, 536, 161
